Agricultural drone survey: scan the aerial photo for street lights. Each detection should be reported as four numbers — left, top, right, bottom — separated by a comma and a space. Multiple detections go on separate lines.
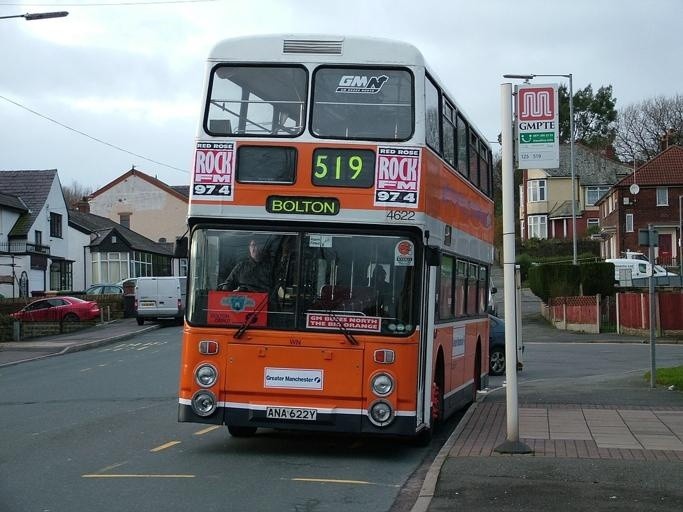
504, 74, 577, 264
0, 11, 68, 21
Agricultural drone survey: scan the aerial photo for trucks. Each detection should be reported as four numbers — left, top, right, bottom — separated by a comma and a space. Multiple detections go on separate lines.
134, 276, 187, 326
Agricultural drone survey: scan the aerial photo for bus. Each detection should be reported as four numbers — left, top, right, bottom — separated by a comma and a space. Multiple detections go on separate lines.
176, 32, 497, 449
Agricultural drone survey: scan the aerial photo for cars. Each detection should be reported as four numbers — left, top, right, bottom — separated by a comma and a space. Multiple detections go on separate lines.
485, 277, 499, 317
487, 313, 524, 376
9, 296, 101, 321
604, 258, 679, 288
621, 252, 650, 262
84, 278, 137, 319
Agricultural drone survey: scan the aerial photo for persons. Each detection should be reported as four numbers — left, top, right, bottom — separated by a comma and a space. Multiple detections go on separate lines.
222, 237, 292, 313
